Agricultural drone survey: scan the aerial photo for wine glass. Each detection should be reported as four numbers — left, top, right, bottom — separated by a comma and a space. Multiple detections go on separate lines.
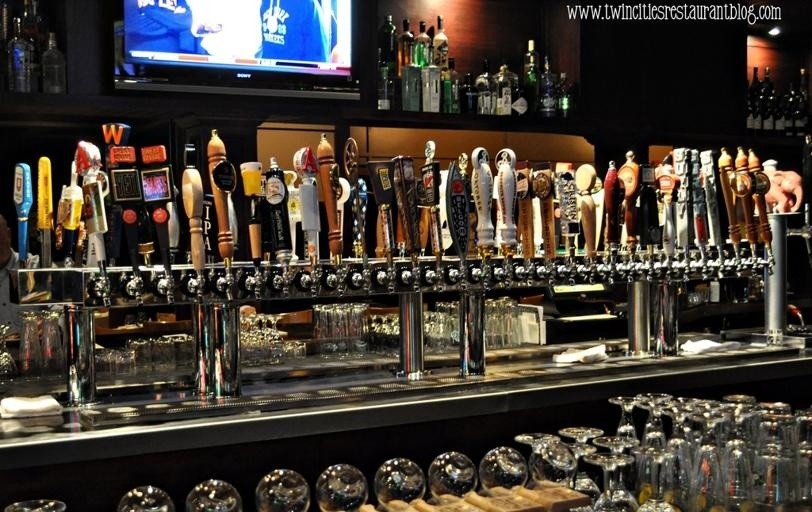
513, 392, 812, 511
1, 323, 18, 379
239, 310, 306, 367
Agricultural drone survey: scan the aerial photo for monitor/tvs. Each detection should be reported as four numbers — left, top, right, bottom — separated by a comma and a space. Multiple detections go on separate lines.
112, 0, 361, 104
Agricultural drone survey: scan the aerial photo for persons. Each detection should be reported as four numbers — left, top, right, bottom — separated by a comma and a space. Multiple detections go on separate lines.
127, 0, 222, 54
256, 0, 338, 60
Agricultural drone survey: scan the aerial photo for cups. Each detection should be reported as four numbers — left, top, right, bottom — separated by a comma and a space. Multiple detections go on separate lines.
22, 310, 63, 375
688, 291, 704, 305
423, 295, 523, 352
370, 312, 400, 337
313, 302, 372, 358
93, 330, 196, 377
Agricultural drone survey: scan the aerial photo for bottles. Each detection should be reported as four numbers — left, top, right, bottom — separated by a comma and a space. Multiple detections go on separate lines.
745, 62, 811, 138
0, 0, 69, 99
709, 280, 720, 303
364, 12, 578, 119
